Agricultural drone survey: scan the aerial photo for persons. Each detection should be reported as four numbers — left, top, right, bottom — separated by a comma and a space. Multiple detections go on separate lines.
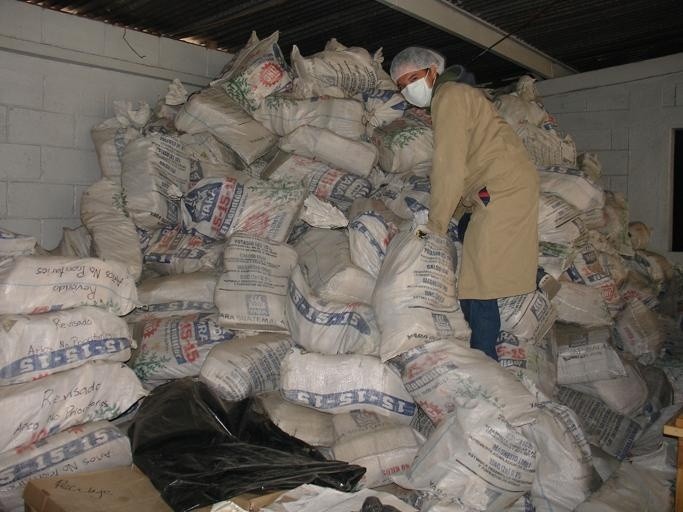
390, 47, 561, 361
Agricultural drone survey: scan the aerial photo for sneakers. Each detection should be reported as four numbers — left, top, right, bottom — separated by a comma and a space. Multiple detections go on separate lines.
536, 269, 561, 299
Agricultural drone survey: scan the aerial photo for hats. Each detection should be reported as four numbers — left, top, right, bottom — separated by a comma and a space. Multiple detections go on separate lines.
390, 47, 445, 82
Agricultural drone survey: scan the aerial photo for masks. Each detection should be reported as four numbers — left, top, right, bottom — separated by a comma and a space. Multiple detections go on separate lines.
401, 70, 436, 107
